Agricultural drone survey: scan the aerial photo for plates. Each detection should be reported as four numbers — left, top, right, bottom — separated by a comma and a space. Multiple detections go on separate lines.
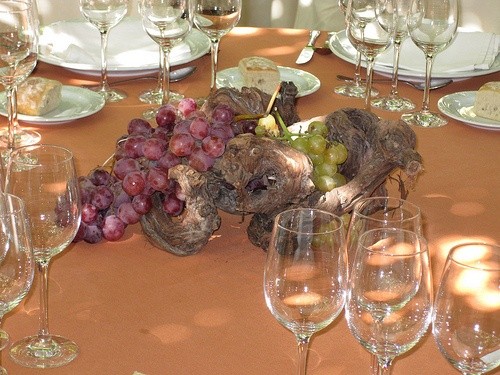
0, 83, 105, 126
34, 15, 211, 76
328, 29, 500, 82
437, 91, 500, 131
215, 67, 322, 99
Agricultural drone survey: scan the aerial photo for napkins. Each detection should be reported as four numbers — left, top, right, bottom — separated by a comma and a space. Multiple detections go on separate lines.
336, 31, 500, 72
44, 17, 191, 65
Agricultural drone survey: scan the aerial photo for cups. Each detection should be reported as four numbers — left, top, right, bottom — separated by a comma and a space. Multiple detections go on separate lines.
432, 243, 500, 375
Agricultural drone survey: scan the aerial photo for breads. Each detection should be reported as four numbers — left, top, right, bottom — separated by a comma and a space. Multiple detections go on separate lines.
475, 81, 500, 121
239, 56, 281, 96
0, 76, 62, 116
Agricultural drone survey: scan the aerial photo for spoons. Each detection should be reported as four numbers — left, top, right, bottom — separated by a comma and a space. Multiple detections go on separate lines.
336, 74, 453, 90
80, 66, 197, 88
313, 30, 338, 56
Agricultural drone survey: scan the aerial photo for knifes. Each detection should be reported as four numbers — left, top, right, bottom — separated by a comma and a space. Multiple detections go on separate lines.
296, 30, 320, 64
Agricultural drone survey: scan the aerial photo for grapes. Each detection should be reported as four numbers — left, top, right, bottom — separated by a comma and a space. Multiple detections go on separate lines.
254, 118, 349, 192
56, 97, 258, 243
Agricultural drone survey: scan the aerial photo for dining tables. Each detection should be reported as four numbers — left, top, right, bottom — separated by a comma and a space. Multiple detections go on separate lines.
0, 27, 500, 375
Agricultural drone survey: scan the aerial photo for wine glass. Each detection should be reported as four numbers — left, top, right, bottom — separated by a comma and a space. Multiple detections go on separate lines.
263, 208, 349, 375
78, 1, 133, 101
370, 0, 422, 112
0, 0, 82, 374
401, 0, 460, 129
346, 229, 434, 374
138, 0, 186, 121
333, 1, 380, 98
138, 0, 192, 105
345, 1, 391, 124
189, 0, 242, 106
345, 196, 422, 374
